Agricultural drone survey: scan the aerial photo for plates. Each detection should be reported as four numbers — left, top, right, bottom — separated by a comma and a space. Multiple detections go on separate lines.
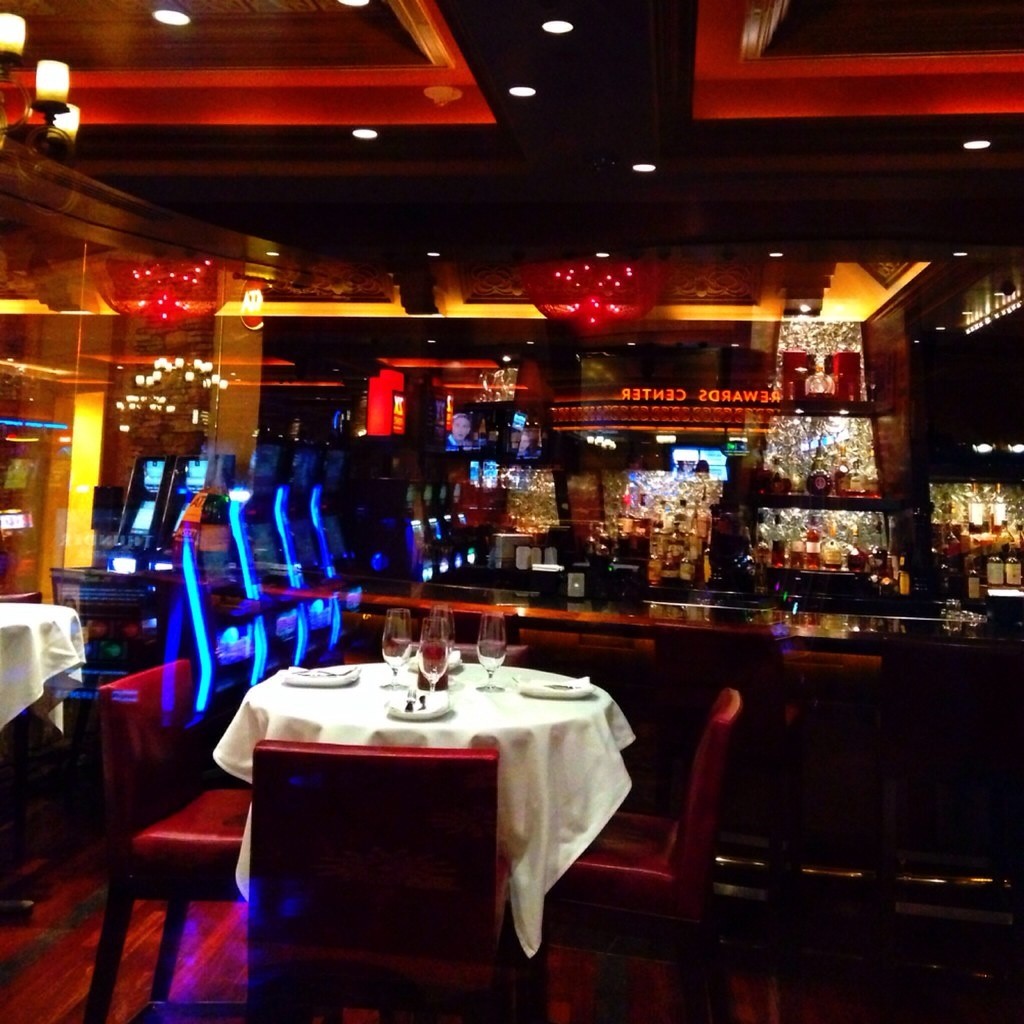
517, 679, 594, 698
385, 696, 452, 720
411, 654, 463, 673
284, 667, 359, 687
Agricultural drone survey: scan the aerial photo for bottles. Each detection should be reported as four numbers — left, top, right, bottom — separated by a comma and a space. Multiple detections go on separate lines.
596, 491, 711, 586
932, 482, 1024, 599
757, 511, 912, 611
765, 437, 878, 496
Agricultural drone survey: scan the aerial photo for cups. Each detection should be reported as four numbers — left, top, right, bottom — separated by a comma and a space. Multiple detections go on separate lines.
428, 604, 454, 655
941, 600, 962, 631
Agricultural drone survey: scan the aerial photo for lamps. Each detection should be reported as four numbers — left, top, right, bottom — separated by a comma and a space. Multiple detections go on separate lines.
152, 0, 192, 26
0, 13, 81, 226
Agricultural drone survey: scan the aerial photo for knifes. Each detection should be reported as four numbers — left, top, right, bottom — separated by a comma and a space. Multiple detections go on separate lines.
419, 695, 426, 710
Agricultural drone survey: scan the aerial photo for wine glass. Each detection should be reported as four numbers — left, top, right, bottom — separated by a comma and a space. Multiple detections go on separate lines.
476, 609, 507, 694
382, 609, 412, 692
418, 618, 449, 707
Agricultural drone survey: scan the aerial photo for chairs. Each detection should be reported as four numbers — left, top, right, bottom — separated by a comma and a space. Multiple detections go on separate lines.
246, 741, 509, 1024
82, 659, 256, 1024
511, 685, 743, 1024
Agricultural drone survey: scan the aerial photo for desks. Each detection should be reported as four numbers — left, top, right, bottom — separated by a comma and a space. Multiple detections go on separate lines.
0, 602, 87, 860
209, 659, 637, 1024
